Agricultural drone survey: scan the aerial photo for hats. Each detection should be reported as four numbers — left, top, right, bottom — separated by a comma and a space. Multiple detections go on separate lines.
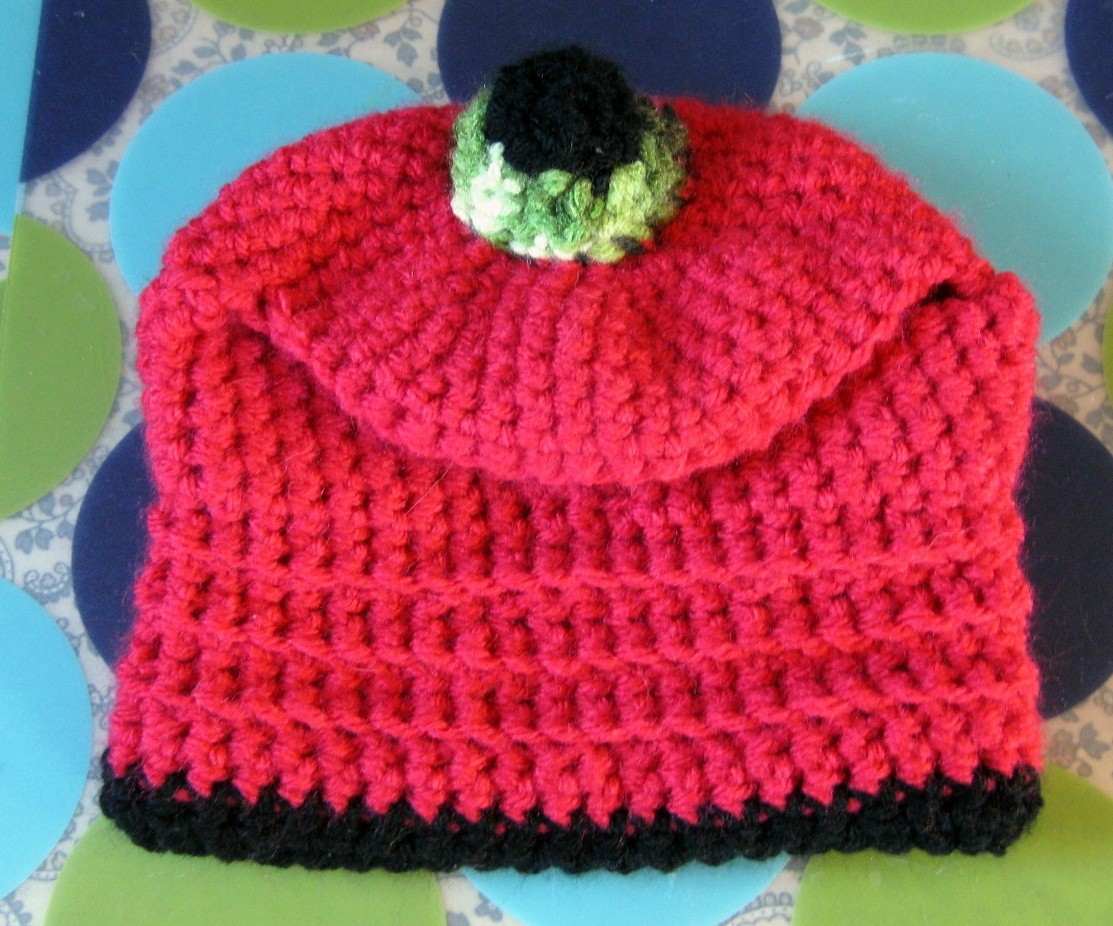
101, 41, 1045, 875
